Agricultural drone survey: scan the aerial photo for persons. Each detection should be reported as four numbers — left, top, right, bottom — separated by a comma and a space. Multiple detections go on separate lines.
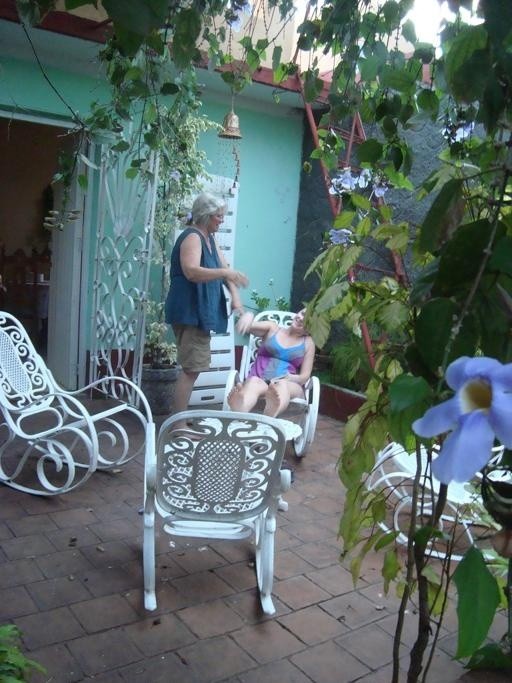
227, 306, 315, 417
165, 192, 249, 440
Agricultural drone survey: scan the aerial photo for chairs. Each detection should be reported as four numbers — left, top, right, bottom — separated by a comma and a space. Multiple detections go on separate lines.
223, 310, 321, 458
368, 441, 512, 562
0, 310, 153, 497
142, 410, 291, 616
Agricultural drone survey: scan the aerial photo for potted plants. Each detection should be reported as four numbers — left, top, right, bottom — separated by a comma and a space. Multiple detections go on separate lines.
131, 97, 226, 416
